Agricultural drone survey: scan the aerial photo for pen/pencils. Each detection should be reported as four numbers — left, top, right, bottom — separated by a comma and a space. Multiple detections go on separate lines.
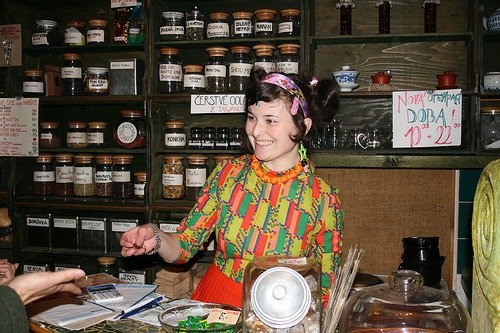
122, 296, 155, 314
120, 297, 163, 319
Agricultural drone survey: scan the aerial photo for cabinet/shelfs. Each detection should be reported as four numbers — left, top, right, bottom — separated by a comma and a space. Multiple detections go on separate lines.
0, 0, 500, 275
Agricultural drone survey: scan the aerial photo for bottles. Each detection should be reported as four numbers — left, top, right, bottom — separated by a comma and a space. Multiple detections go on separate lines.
157, 0, 299, 95
479, 106, 500, 149
84, 67, 109, 95
486, 4, 500, 31
112, 110, 145, 149
87, 122, 107, 148
97, 256, 116, 278
484, 71, 500, 91
33, 152, 146, 200
31, 4, 144, 46
161, 120, 245, 199
243, 254, 463, 333
66, 122, 87, 148
310, 118, 392, 152
39, 121, 60, 147
22, 69, 46, 96
59, 53, 84, 96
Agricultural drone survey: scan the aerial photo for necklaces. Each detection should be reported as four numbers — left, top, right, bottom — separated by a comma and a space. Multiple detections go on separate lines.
251, 154, 304, 185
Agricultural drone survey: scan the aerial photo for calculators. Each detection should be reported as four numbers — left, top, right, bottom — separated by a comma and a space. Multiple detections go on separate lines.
86, 283, 124, 303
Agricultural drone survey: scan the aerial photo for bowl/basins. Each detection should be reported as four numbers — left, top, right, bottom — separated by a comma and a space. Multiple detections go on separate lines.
158, 302, 243, 333
399, 235, 446, 288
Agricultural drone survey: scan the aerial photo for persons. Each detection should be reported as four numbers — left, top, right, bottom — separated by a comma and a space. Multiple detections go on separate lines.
0, 259, 86, 333
120, 67, 344, 325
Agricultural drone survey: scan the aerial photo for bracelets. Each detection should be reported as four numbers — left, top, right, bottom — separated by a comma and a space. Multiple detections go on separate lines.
146, 223, 161, 255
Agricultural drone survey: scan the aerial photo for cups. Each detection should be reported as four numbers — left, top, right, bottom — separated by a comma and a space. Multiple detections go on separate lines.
370, 71, 392, 84
436, 70, 459, 90
333, 66, 358, 92
